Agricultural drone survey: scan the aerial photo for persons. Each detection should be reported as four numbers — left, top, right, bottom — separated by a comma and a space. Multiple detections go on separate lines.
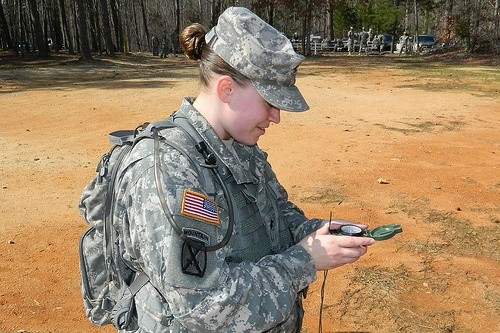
359, 26, 368, 55
79, 5, 376, 333
366, 28, 385, 52
346, 22, 358, 57
397, 30, 410, 58
290, 30, 344, 53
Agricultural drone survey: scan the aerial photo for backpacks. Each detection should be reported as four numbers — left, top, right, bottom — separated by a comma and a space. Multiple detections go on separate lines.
78, 120, 217, 330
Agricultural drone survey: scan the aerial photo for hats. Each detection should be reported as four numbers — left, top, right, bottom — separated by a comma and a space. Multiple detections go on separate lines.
205, 6, 310, 112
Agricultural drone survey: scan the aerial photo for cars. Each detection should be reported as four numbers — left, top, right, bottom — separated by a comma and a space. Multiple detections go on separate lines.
378, 34, 392, 50
293, 31, 372, 52
409, 35, 439, 53
396, 37, 413, 50
